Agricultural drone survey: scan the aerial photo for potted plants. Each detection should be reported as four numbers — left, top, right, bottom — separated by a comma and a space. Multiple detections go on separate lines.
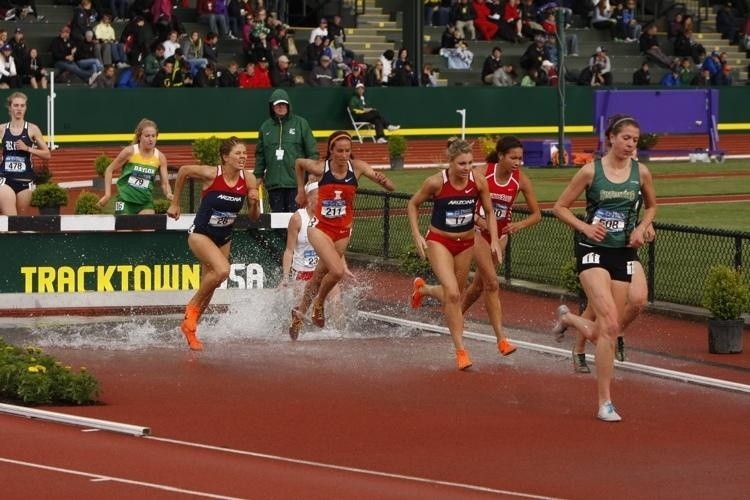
91, 154, 112, 192
636, 132, 658, 162
399, 243, 446, 306
387, 135, 407, 169
701, 263, 750, 353
30, 180, 68, 215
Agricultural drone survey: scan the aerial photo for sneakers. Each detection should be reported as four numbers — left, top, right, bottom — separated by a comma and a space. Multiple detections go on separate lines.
311, 297, 325, 327
377, 137, 388, 144
552, 305, 626, 422
184, 301, 201, 332
180, 323, 201, 351
496, 339, 517, 356
455, 349, 472, 370
387, 125, 400, 130
410, 277, 426, 308
288, 306, 304, 339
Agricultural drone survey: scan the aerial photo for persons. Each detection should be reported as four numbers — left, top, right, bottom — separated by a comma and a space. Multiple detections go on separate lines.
280, 177, 354, 327
456, 137, 544, 358
0, 90, 56, 217
288, 127, 399, 340
165, 137, 263, 352
1, 1, 750, 87
571, 221, 658, 378
96, 117, 175, 234
548, 111, 659, 427
405, 134, 507, 374
347, 83, 403, 146
253, 88, 319, 213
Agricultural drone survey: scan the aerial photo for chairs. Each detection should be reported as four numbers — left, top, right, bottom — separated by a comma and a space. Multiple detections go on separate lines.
344, 106, 376, 145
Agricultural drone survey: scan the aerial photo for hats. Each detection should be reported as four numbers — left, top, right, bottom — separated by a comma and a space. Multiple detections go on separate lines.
356, 83, 364, 88
0, 27, 24, 52
278, 55, 289, 63
321, 55, 330, 61
